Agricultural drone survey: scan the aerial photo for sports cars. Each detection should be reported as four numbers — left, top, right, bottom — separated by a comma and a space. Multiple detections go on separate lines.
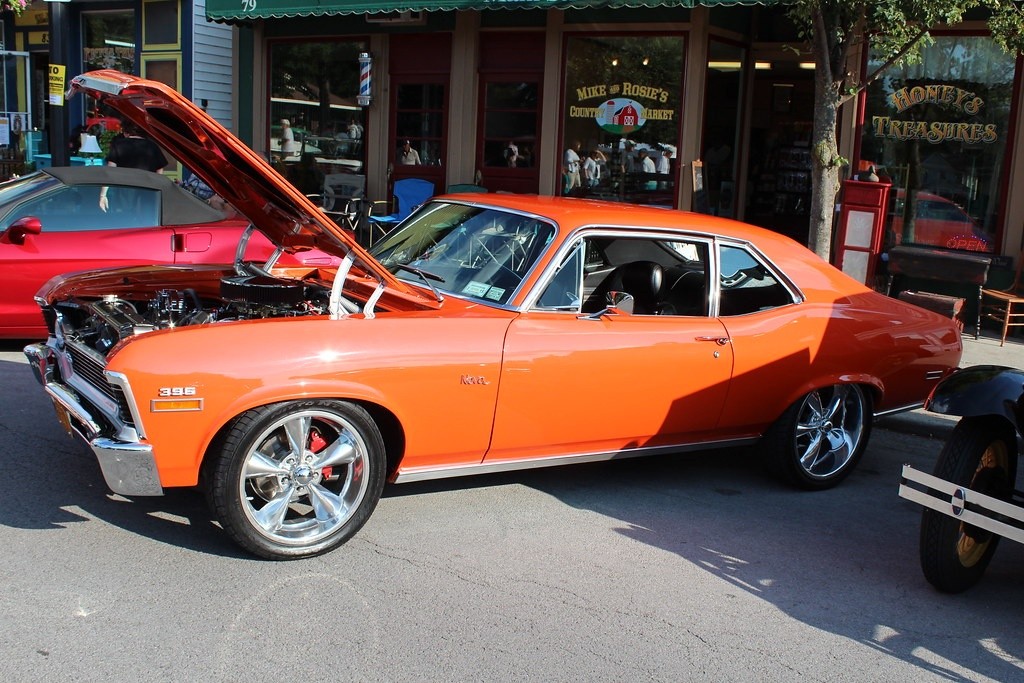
24, 69, 963, 561
0, 166, 355, 338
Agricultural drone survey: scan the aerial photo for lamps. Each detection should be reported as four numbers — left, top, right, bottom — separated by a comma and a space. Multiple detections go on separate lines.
79, 132, 102, 166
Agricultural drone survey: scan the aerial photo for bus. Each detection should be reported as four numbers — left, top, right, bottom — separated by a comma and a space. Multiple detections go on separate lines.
266, 98, 363, 157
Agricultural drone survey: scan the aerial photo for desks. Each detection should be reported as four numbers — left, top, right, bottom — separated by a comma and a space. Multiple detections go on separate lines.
887, 243, 995, 341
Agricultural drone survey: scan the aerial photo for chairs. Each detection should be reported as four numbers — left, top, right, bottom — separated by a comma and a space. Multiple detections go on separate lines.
981, 248, 1024, 346
469, 190, 538, 272
422, 184, 489, 265
582, 261, 667, 313
303, 173, 366, 246
368, 178, 436, 249
523, 241, 581, 308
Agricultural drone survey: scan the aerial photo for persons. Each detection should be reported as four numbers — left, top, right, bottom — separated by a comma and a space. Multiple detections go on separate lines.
99, 120, 168, 213
277, 118, 295, 158
564, 138, 673, 196
400, 140, 421, 165
508, 141, 524, 168
323, 120, 363, 154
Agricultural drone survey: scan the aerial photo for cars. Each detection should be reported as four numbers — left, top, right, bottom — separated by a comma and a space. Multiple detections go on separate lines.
271, 126, 322, 161
891, 188, 998, 253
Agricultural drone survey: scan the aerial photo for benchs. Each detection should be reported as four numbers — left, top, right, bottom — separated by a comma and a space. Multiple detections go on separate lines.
900, 289, 968, 333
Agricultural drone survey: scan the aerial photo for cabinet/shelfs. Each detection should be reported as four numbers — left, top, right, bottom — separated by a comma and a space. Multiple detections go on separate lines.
752, 144, 811, 220
